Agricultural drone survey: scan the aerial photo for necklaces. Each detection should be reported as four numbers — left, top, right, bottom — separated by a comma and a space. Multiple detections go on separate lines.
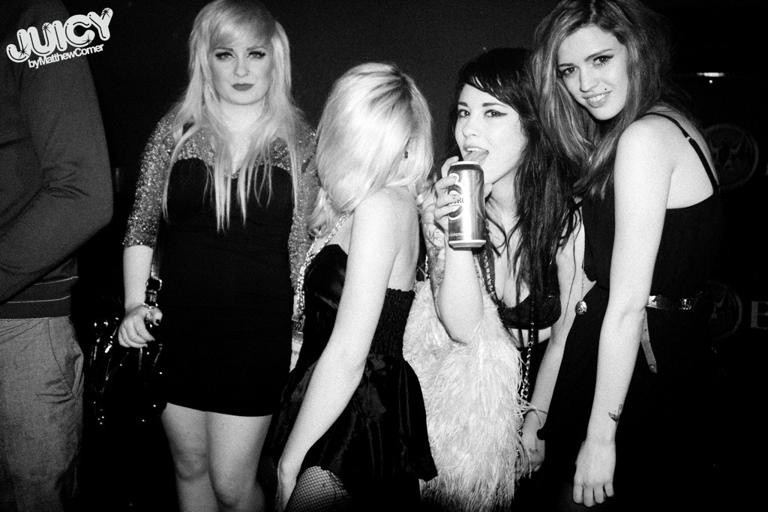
482, 249, 536, 406
296, 214, 348, 333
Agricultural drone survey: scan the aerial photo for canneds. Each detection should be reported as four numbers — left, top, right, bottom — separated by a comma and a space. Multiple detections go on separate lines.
448, 162, 486, 251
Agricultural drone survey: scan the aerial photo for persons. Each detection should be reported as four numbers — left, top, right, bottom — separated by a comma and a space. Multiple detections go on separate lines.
421, 46, 596, 512
255, 63, 438, 511
2, 0, 115, 509
535, 1, 720, 512
117, 0, 320, 512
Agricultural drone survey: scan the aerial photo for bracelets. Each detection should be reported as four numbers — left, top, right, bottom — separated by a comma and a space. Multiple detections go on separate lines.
524, 403, 548, 428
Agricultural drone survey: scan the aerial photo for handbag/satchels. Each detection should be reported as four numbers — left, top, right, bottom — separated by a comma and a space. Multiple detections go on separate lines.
83, 272, 172, 447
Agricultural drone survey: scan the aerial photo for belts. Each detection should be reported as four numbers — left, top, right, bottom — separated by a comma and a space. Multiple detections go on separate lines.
645, 295, 692, 311
